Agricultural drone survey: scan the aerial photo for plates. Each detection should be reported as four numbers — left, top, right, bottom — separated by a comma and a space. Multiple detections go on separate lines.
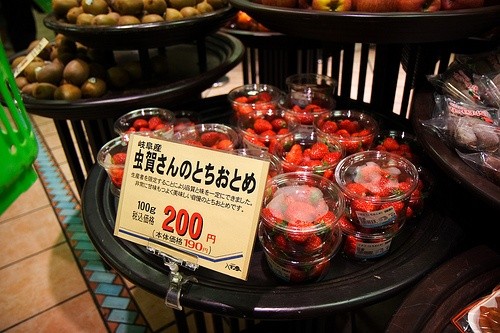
383, 241, 500, 333
227, 1, 500, 42
411, 79, 500, 212
0, 32, 245, 120
80, 101, 466, 319
220, 28, 311, 45
43, 2, 234, 49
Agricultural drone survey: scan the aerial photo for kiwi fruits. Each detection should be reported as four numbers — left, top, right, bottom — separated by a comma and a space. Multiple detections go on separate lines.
10, 34, 105, 101
50, 0, 223, 27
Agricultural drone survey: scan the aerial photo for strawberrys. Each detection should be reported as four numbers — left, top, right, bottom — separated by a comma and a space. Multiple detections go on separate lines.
103, 91, 423, 282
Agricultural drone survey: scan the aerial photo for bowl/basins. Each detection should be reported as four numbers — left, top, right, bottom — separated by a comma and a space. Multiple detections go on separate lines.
97, 72, 426, 284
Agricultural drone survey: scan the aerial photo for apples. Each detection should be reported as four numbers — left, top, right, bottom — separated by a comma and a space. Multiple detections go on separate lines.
230, 10, 270, 33
256, 0, 488, 13
